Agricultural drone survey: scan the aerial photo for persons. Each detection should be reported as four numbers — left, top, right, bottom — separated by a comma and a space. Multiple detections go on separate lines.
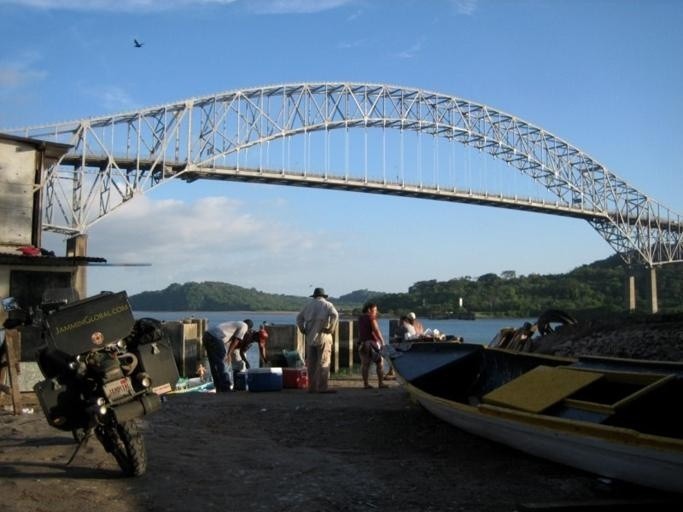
358, 301, 390, 389
384, 311, 424, 380
203, 319, 269, 392
296, 287, 339, 394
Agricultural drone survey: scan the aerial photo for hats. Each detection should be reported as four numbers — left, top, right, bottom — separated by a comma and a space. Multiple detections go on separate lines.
309, 288, 329, 298
259, 329, 269, 347
406, 311, 416, 320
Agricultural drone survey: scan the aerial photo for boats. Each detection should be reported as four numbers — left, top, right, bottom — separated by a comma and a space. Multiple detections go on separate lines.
379, 341, 683, 493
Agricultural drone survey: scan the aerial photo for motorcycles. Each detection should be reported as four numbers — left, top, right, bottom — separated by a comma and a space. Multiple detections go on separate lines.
2, 290, 180, 476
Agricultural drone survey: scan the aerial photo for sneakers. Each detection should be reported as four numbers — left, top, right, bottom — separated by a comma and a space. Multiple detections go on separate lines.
378, 385, 389, 388
364, 384, 373, 389
307, 388, 337, 394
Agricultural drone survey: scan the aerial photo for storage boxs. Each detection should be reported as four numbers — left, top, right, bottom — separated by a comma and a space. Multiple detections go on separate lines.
248, 366, 309, 391
